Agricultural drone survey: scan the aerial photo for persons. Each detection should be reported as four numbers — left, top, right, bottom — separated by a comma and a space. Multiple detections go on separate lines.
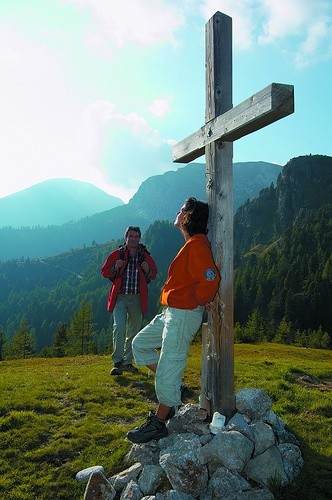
100, 225, 158, 375
125, 197, 221, 444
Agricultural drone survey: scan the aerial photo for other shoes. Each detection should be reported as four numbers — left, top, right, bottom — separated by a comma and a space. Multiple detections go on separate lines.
124, 364, 138, 372
126, 409, 169, 444
110, 364, 122, 376
167, 402, 181, 419
